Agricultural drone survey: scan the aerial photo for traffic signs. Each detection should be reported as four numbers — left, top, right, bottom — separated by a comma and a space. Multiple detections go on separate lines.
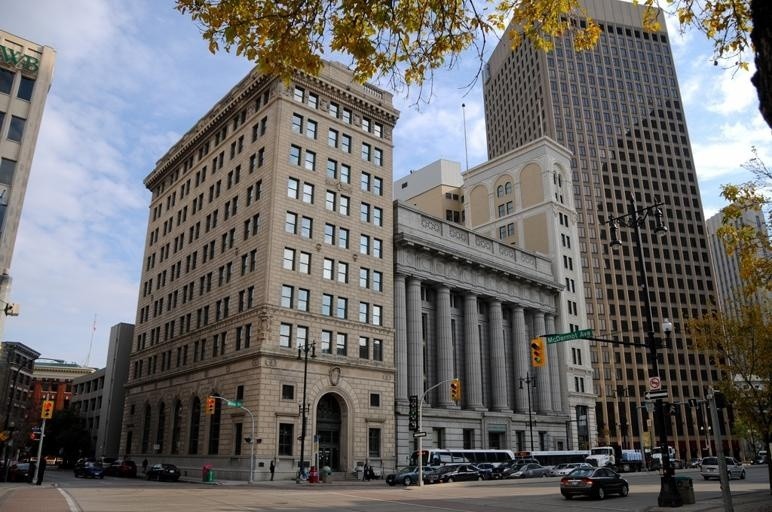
547, 328, 593, 346
227, 400, 243, 408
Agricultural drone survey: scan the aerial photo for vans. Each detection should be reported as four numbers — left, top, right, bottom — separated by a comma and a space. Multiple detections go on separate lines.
699, 456, 747, 478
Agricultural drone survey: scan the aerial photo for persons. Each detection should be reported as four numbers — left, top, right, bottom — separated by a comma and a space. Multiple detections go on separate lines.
139, 455, 151, 475
269, 459, 276, 482
369, 465, 375, 481
364, 463, 369, 478
34, 455, 49, 486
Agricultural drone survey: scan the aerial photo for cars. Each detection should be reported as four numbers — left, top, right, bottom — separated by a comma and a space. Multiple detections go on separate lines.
749, 450, 767, 464
673, 457, 703, 469
8, 462, 38, 482
72, 454, 183, 481
554, 468, 631, 498
385, 458, 594, 484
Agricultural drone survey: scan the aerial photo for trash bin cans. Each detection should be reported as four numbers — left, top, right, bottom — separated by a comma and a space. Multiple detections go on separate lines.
323, 466, 332, 483
203, 465, 214, 482
675, 477, 696, 505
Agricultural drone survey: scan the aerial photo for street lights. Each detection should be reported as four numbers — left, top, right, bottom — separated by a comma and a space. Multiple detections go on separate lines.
2, 355, 70, 488
601, 190, 698, 506
297, 339, 317, 481
699, 425, 712, 456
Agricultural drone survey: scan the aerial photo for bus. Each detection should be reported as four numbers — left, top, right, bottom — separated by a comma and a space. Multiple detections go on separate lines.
410, 441, 677, 466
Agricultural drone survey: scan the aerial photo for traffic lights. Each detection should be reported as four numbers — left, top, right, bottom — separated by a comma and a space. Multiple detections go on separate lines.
27, 431, 35, 440
531, 337, 545, 368
450, 379, 462, 400
41, 400, 54, 419
408, 396, 418, 431
206, 397, 216, 415
